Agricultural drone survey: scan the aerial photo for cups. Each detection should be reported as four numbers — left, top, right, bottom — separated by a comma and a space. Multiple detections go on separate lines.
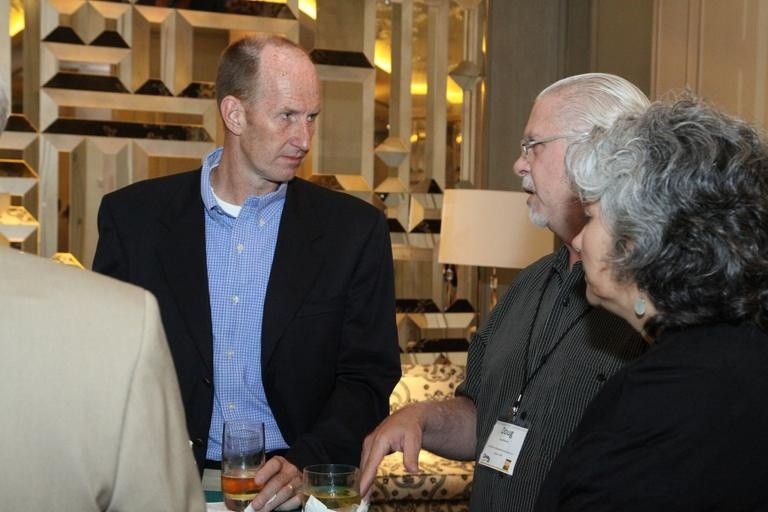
219, 417, 266, 512
301, 462, 362, 512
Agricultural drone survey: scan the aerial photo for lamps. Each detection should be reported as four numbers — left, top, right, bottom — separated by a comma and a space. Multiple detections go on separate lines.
436, 186, 555, 311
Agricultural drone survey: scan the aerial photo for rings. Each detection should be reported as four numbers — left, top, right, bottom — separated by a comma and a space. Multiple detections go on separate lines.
288, 481, 296, 494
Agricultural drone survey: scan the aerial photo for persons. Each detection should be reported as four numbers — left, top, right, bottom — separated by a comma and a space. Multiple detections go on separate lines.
1, 239, 207, 512
534, 85, 767, 512
93, 31, 404, 512
351, 70, 655, 510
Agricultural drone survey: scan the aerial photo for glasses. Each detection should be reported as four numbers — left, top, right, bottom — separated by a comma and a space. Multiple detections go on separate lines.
519, 134, 575, 157
